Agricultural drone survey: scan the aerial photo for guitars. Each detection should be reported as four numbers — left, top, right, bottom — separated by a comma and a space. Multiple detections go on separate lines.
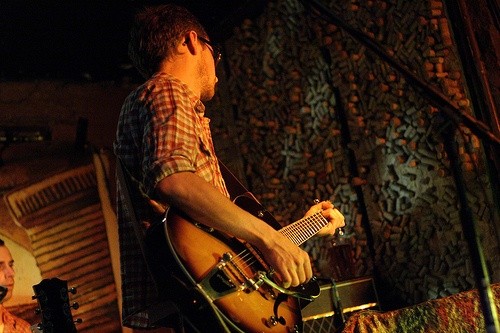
31, 278, 83, 333
133, 199, 344, 333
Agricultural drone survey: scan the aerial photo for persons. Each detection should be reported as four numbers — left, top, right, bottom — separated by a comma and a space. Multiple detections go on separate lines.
0, 238, 35, 333
110, 2, 314, 332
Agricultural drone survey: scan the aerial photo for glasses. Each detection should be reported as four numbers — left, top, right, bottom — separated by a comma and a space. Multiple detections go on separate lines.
183, 36, 221, 65
